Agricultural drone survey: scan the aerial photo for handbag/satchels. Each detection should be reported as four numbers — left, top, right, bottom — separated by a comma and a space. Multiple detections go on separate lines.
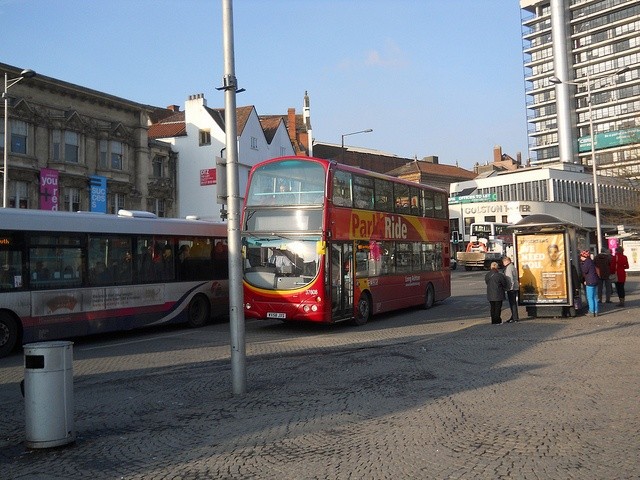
608, 255, 618, 283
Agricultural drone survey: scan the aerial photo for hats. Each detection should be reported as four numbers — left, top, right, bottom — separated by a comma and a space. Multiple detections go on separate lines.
581, 250, 590, 257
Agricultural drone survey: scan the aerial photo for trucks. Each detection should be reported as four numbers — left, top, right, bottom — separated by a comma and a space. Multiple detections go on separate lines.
457, 239, 506, 271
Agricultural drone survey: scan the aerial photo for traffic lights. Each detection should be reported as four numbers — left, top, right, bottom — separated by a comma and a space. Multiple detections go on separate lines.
453, 232, 458, 243
459, 234, 462, 240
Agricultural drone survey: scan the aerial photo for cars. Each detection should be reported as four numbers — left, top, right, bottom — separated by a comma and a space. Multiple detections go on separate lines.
450, 258, 457, 270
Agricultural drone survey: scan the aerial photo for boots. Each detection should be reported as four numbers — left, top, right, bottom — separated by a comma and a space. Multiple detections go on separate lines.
616, 298, 625, 307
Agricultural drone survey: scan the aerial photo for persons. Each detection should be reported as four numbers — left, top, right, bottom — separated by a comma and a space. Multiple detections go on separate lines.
484, 261, 507, 324
498, 256, 519, 323
577, 250, 601, 318
593, 246, 614, 304
545, 245, 564, 269
609, 246, 630, 308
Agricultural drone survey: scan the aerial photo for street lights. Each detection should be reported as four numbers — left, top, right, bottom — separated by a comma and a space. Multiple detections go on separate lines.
341, 129, 373, 164
548, 71, 602, 254
1, 68, 36, 208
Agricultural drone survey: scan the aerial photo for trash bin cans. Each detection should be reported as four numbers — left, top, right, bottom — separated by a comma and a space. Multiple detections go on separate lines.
20, 340, 76, 449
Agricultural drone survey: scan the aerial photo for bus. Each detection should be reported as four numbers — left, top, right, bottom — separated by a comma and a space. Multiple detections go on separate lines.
240, 156, 451, 326
0, 207, 229, 359
470, 222, 513, 245
